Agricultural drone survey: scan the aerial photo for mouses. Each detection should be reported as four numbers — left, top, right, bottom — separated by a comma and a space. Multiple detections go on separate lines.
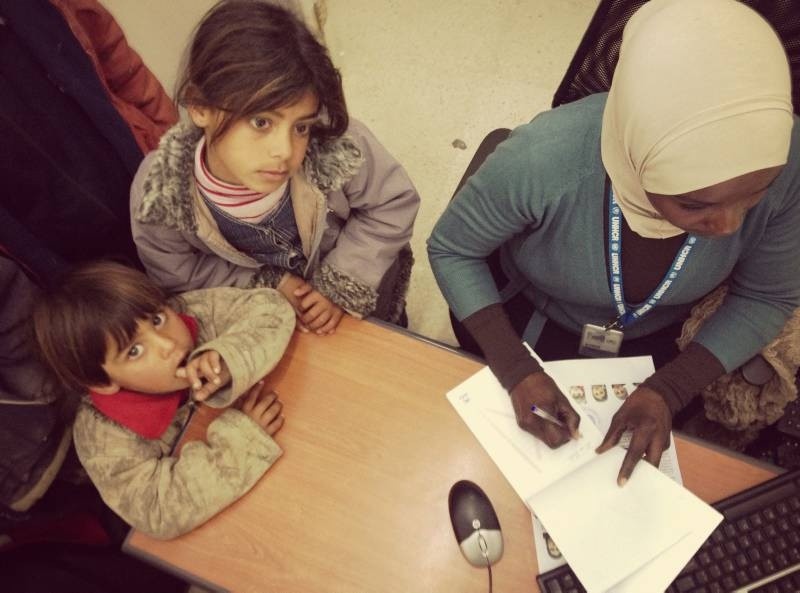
448, 481, 503, 567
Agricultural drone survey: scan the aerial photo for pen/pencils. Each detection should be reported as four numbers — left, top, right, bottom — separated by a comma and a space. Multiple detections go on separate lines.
531, 405, 582, 438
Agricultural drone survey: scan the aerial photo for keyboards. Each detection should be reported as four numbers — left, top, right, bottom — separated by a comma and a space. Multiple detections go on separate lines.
534, 467, 800, 593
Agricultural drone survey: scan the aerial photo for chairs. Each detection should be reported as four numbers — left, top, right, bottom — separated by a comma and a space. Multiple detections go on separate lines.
447, 0, 800, 432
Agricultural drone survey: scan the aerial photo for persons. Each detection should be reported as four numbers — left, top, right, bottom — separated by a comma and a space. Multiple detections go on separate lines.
569, 384, 628, 401
130, 0, 421, 337
0, 0, 181, 514
33, 259, 295, 542
425, 0, 800, 487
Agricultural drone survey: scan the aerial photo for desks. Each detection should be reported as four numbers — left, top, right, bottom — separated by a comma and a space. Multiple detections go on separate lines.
122, 313, 786, 593
